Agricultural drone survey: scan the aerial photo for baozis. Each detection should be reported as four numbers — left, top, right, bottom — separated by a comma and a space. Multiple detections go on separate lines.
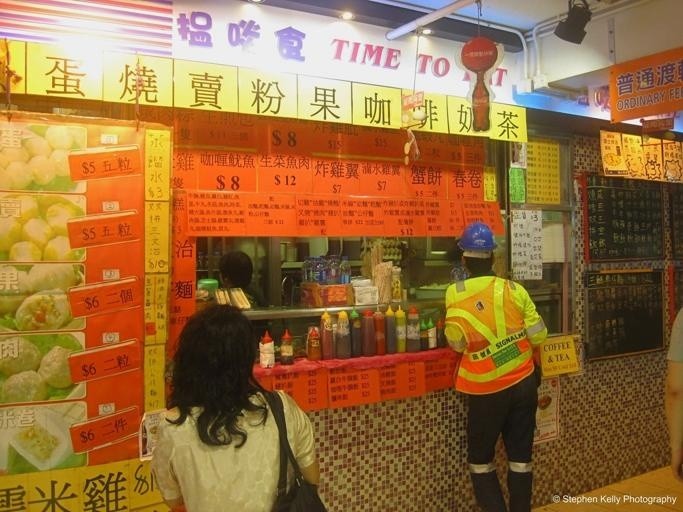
0, 125, 77, 402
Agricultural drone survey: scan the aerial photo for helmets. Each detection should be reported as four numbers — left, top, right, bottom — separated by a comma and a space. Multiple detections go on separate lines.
457, 221, 499, 259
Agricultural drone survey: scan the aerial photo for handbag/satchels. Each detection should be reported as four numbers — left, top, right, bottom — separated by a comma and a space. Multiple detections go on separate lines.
259, 388, 328, 511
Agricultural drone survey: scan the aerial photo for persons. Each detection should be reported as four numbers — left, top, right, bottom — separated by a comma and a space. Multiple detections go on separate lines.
663, 305, 683, 489
441, 221, 549, 512
148, 305, 321, 512
210, 250, 258, 308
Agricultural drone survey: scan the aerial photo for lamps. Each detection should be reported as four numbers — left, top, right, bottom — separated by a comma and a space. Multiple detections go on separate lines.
553, 0, 594, 44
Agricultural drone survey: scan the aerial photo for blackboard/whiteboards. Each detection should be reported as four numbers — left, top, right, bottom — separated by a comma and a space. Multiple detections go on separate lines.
582, 171, 683, 362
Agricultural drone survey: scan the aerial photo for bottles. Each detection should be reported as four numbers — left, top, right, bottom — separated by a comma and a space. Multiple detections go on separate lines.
471, 78, 493, 132
303, 254, 352, 284
260, 305, 447, 370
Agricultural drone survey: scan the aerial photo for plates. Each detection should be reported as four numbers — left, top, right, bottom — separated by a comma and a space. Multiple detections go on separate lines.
8, 415, 68, 471
